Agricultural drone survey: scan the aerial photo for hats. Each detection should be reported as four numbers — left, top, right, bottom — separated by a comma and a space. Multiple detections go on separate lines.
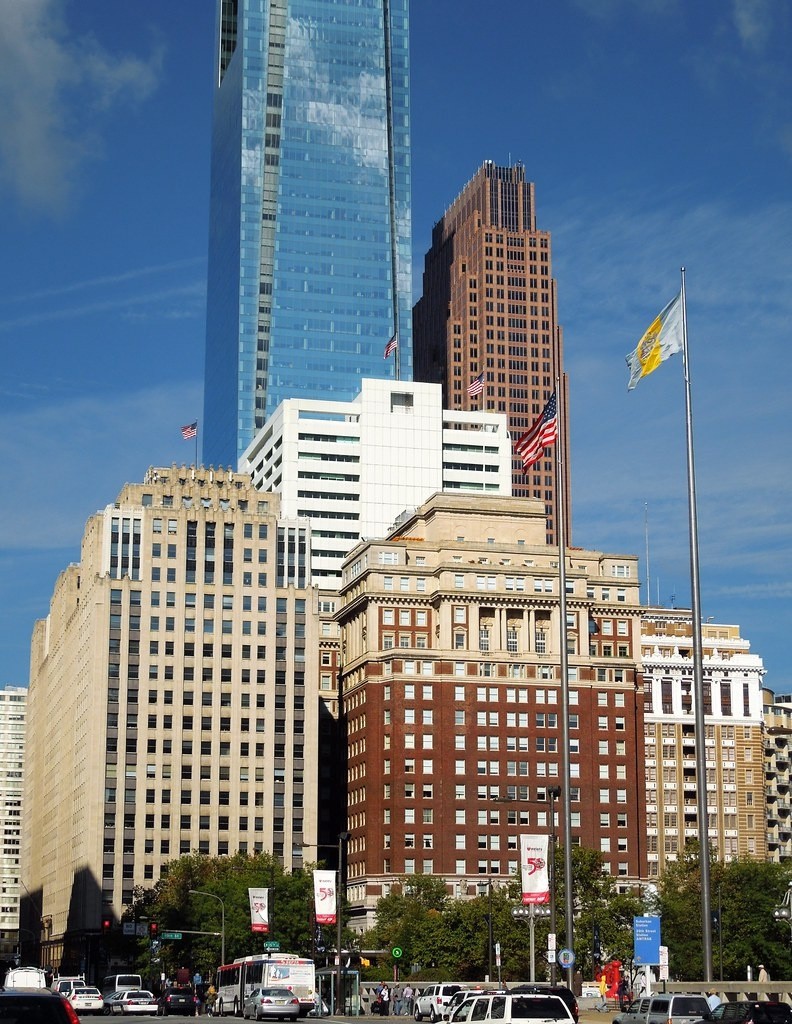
395, 983, 399, 986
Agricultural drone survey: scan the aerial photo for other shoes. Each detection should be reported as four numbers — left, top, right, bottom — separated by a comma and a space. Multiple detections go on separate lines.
605, 1010, 611, 1013
595, 1007, 600, 1012
404, 1012, 408, 1015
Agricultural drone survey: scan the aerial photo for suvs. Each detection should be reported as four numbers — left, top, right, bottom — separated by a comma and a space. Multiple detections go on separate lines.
158, 986, 196, 1016
414, 982, 579, 1024
612, 994, 792, 1024
0, 986, 82, 1024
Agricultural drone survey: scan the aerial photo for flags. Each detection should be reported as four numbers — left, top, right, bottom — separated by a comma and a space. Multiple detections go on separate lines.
466, 371, 485, 397
384, 335, 396, 359
514, 390, 558, 476
625, 291, 684, 392
181, 422, 197, 440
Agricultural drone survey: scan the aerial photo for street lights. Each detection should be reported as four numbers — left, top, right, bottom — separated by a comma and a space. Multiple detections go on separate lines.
477, 879, 494, 982
492, 783, 562, 986
296, 831, 352, 1016
188, 890, 225, 966
511, 906, 551, 982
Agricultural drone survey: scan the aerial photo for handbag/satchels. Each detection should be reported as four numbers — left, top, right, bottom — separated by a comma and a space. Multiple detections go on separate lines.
378, 993, 381, 1003
617, 987, 622, 995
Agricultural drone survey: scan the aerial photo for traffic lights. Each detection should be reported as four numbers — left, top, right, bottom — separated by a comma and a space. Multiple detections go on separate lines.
102, 917, 113, 935
150, 921, 157, 940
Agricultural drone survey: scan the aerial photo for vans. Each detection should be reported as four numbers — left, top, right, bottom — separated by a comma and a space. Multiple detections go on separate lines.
51, 974, 86, 998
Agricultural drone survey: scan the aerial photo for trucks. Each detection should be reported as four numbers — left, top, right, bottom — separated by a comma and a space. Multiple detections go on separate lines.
4, 967, 48, 988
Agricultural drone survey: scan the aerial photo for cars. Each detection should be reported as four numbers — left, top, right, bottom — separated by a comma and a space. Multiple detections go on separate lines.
65, 986, 104, 1016
104, 990, 159, 1016
243, 987, 300, 1021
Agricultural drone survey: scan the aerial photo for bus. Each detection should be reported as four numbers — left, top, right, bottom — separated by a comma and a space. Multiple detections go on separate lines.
95, 975, 142, 997
212, 953, 316, 1018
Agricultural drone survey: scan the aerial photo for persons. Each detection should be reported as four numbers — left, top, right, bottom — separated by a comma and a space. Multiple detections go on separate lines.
204, 986, 218, 1017
574, 971, 582, 996
403, 983, 413, 1016
640, 972, 646, 993
758, 965, 768, 982
393, 983, 404, 1015
596, 976, 611, 1013
377, 981, 390, 1016
618, 975, 631, 1008
